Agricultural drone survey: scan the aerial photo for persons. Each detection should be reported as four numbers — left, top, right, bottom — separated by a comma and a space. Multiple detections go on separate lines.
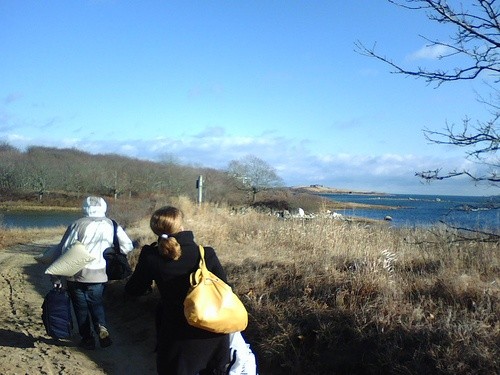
125, 206, 230, 375
62, 196, 134, 349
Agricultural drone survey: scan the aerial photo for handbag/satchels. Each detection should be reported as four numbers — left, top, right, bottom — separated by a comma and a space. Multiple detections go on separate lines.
102, 220, 132, 281
40, 282, 74, 344
224, 330, 257, 375
183, 245, 250, 336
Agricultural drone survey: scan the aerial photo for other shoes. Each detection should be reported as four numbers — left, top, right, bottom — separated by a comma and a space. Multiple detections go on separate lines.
96, 324, 112, 347
78, 336, 96, 350
